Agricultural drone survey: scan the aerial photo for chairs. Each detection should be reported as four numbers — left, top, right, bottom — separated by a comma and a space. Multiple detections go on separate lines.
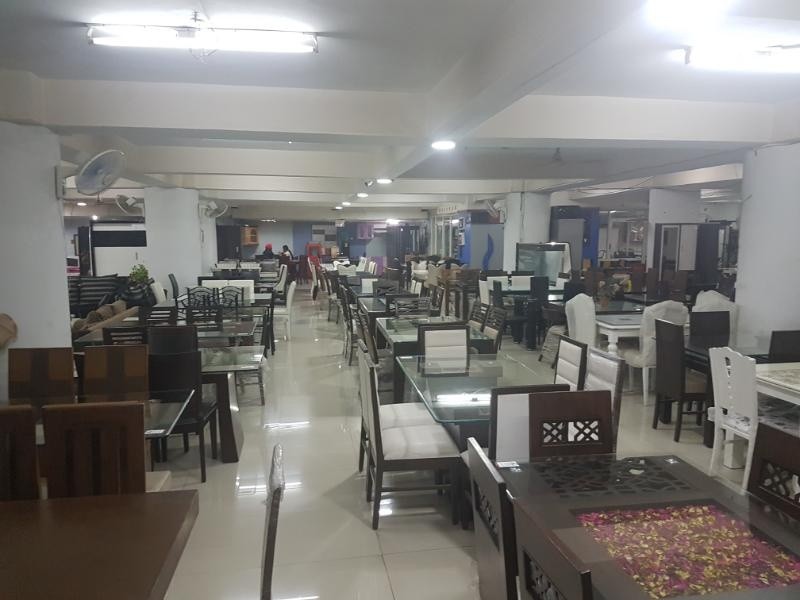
0, 256, 800, 600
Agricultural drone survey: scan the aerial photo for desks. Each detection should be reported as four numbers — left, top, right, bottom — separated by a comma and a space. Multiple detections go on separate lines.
499, 455, 800, 600
489, 286, 565, 350
0, 388, 194, 437
0, 489, 199, 600
160, 320, 254, 346
651, 335, 769, 450
595, 314, 642, 355
376, 316, 495, 404
74, 345, 266, 463
398, 354, 554, 531
723, 362, 800, 468
350, 285, 406, 304
359, 296, 428, 341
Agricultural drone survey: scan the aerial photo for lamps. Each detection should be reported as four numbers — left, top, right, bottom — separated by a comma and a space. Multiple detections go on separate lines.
85, 21, 320, 55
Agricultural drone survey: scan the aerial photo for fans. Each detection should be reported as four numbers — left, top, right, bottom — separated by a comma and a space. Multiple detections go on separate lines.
199, 198, 228, 218
55, 150, 126, 198
486, 199, 508, 221
115, 194, 144, 218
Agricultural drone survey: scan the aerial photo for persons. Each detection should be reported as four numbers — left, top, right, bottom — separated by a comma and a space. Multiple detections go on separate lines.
619, 272, 631, 292
263, 242, 274, 255
281, 245, 293, 260
599, 267, 620, 298
